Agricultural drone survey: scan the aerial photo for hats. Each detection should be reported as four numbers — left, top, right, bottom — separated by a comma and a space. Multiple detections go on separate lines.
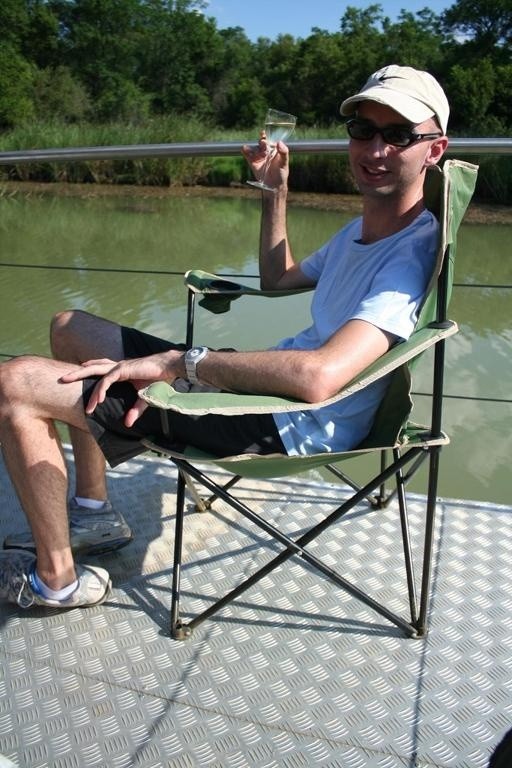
338, 64, 450, 136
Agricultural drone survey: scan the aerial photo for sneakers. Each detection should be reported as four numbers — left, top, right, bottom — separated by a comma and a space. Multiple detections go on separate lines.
4, 497, 135, 559
0, 549, 113, 608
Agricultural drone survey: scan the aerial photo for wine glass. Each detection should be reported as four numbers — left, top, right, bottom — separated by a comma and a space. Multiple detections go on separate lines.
247, 108, 298, 194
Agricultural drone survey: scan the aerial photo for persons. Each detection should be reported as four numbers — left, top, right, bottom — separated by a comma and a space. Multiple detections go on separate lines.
0, 64, 451, 613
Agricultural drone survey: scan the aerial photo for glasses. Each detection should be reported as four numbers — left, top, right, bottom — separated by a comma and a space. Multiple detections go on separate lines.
345, 118, 441, 148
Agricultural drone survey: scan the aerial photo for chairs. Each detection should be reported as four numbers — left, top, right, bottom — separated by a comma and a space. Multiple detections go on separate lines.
131, 155, 483, 641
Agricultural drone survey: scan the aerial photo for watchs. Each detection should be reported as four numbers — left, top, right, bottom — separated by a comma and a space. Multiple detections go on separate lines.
182, 346, 208, 388
